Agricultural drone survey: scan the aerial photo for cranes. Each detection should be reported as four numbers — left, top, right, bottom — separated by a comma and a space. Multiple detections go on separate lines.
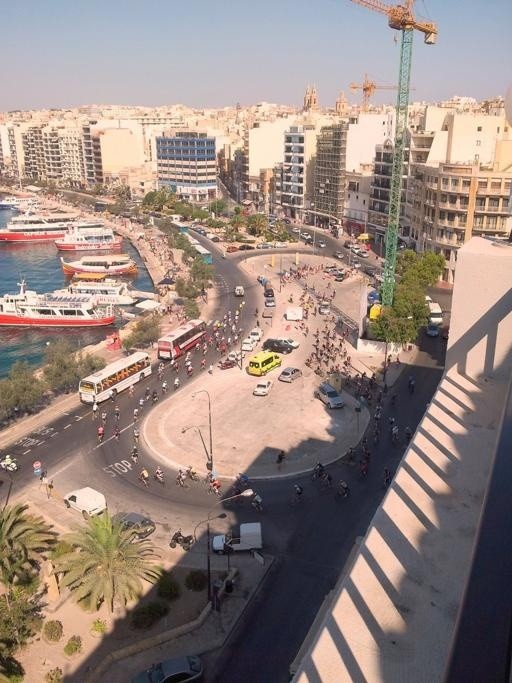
347, 70, 418, 114
335, 0, 440, 311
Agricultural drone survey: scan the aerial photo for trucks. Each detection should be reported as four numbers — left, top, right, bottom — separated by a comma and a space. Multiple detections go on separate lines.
63, 484, 106, 519
210, 520, 264, 554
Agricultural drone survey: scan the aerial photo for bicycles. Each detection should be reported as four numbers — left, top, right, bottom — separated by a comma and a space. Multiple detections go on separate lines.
286, 406, 411, 506
134, 464, 271, 514
84, 405, 146, 464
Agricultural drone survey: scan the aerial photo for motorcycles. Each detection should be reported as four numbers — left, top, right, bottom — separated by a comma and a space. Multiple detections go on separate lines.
1, 457, 19, 473
169, 527, 194, 550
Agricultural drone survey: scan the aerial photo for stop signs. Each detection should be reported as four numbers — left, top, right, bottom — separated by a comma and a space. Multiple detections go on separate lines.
34, 461, 41, 469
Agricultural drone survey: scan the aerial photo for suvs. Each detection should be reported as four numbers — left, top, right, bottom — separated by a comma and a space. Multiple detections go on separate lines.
111, 508, 157, 539
315, 383, 345, 410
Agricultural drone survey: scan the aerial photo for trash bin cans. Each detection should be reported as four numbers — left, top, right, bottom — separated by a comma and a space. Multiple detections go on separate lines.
226, 580, 233, 593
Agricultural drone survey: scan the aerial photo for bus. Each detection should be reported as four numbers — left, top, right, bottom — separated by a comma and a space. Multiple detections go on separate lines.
178, 231, 200, 245
190, 244, 213, 263
170, 220, 189, 232
157, 319, 208, 361
79, 351, 152, 405
122, 204, 140, 216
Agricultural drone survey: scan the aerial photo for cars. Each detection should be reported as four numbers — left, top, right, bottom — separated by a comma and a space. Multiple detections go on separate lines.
190, 225, 210, 234
227, 245, 238, 252
257, 242, 273, 248
144, 208, 164, 218
130, 653, 205, 682
221, 273, 302, 398
422, 292, 448, 341
318, 305, 331, 315
267, 214, 398, 281
239, 244, 255, 249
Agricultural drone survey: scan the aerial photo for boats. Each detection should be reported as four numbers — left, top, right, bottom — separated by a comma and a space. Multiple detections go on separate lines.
0, 196, 136, 326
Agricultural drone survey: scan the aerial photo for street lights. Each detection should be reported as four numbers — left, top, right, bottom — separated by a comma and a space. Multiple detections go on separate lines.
191, 389, 213, 473
205, 484, 258, 602
